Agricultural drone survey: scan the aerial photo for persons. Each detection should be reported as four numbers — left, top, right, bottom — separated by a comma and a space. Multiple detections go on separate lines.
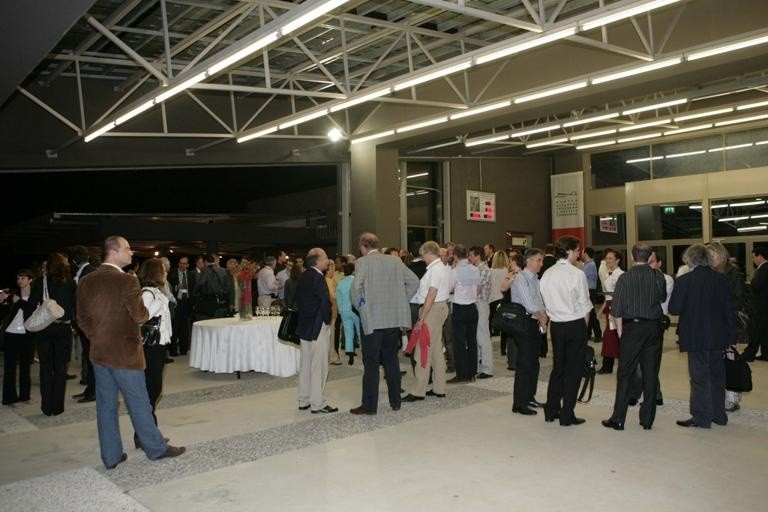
667, 244, 742, 431
601, 241, 668, 431
648, 249, 674, 406
159, 250, 307, 364
539, 235, 595, 427
1, 245, 95, 415
705, 243, 768, 413
293, 231, 557, 415
575, 247, 624, 374
76, 234, 186, 469
134, 257, 170, 451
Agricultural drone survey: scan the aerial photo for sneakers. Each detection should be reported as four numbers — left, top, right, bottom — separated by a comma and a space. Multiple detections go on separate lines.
332, 360, 342, 365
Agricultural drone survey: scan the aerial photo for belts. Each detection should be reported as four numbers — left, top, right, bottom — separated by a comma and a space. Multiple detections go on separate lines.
624, 318, 660, 322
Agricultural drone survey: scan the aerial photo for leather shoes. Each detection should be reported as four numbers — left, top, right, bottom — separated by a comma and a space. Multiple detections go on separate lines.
111, 438, 185, 470
299, 372, 493, 413
66, 374, 96, 403
513, 395, 738, 430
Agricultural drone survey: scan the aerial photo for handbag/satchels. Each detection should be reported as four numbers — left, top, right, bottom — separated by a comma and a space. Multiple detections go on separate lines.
278, 308, 300, 345
24, 300, 64, 332
582, 346, 596, 378
490, 302, 527, 336
141, 315, 161, 346
724, 357, 752, 392
197, 295, 217, 316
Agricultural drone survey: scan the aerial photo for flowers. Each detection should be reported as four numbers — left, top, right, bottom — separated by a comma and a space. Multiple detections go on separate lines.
234, 257, 263, 318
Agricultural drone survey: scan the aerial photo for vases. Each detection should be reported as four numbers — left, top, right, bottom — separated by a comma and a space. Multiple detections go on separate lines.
237, 279, 254, 320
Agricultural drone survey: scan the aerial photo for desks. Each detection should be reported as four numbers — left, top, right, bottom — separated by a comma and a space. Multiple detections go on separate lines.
192, 317, 298, 379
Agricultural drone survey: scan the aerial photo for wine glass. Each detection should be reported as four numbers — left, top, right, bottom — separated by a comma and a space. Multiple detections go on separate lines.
254, 304, 281, 320
